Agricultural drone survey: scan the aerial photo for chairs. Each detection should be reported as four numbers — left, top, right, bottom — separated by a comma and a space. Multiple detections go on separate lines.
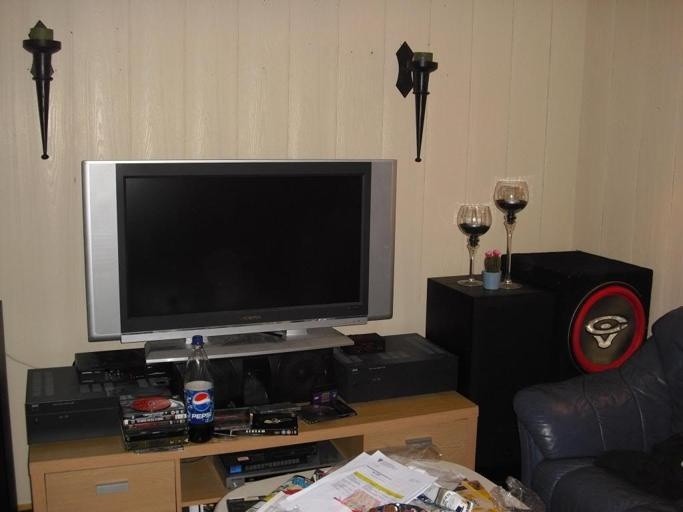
513, 305, 682, 512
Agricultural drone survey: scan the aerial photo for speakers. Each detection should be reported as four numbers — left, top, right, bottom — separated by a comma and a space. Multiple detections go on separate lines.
425, 272, 557, 489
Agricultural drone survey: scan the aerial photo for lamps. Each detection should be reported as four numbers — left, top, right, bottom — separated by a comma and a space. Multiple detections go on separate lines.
22, 19, 61, 160
396, 40, 436, 162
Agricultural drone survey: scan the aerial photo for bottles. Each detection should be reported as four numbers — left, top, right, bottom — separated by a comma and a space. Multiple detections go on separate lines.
182, 334, 216, 444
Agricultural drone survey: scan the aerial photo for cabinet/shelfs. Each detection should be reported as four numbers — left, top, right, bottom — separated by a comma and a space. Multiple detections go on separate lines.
28, 390, 479, 512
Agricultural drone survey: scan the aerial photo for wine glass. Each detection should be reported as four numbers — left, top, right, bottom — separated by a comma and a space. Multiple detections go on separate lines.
492, 177, 528, 291
454, 202, 492, 288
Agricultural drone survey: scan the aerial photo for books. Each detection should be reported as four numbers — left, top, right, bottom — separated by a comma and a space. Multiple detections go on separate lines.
248, 449, 439, 512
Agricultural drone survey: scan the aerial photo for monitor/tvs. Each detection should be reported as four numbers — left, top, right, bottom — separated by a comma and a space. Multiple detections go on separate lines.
80, 158, 398, 365
